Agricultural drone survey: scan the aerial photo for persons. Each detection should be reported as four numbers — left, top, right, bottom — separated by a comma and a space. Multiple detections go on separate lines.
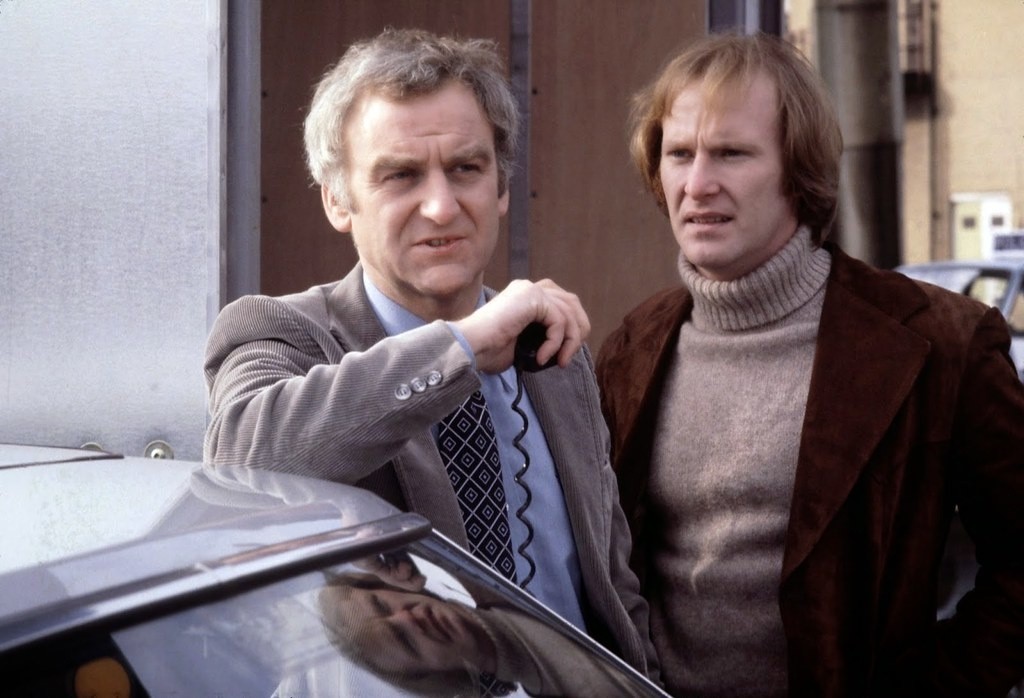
203, 31, 663, 687
594, 33, 1024, 698
318, 550, 646, 698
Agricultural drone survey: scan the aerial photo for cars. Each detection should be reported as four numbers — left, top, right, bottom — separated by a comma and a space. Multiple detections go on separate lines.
0, 439, 675, 698
889, 259, 1024, 384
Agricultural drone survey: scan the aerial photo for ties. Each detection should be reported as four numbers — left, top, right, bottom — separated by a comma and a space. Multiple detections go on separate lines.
438, 390, 517, 584
